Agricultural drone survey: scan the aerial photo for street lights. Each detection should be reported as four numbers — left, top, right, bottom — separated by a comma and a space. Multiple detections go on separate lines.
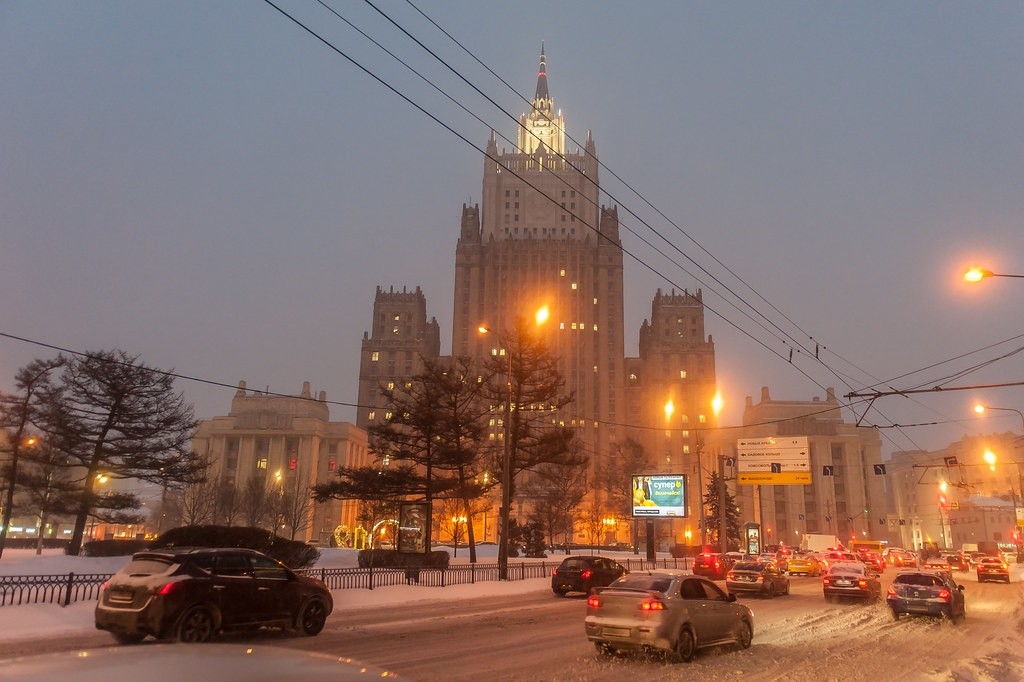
664, 400, 726, 553
479, 307, 549, 581
975, 406, 1024, 432
985, 452, 1024, 508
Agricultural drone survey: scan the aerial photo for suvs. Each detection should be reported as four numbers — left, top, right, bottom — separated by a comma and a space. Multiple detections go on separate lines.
693, 552, 735, 580
95, 547, 333, 644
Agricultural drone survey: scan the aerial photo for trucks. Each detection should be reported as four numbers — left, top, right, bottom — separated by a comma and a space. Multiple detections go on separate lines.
799, 534, 851, 556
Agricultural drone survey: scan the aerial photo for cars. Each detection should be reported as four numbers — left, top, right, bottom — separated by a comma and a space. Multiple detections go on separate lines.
823, 561, 882, 604
584, 572, 755, 664
886, 569, 966, 623
552, 555, 630, 597
725, 558, 791, 598
723, 544, 1024, 568
977, 556, 1010, 584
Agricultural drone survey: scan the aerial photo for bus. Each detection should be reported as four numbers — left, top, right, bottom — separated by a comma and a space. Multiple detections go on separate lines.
849, 540, 888, 556
